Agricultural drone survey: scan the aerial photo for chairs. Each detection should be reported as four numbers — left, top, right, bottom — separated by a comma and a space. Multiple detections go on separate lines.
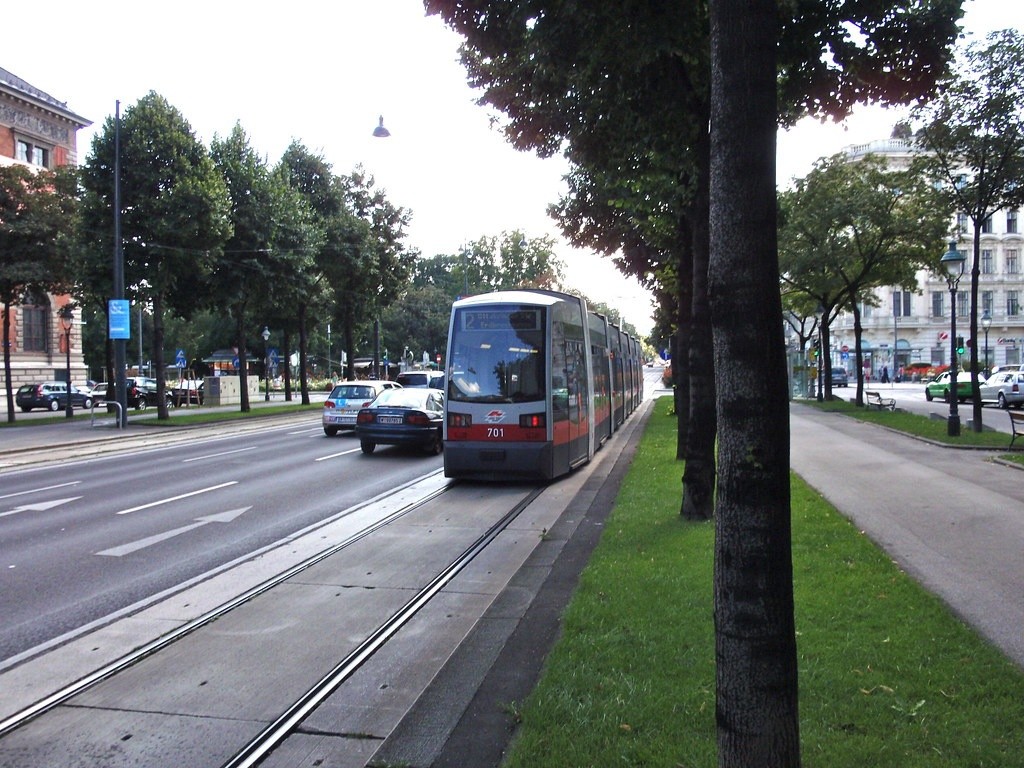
356, 388, 372, 398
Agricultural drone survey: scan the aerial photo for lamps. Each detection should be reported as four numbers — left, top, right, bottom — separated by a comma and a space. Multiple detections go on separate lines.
373, 114, 391, 137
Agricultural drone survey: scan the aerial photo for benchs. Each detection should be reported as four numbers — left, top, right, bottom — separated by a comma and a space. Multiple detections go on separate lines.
866, 391, 896, 411
1005, 410, 1024, 451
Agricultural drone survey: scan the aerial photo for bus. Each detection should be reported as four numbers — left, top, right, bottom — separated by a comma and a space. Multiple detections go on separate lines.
442, 288, 644, 486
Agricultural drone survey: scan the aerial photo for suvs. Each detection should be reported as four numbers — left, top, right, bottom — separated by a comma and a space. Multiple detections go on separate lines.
16, 381, 99, 412
113, 376, 175, 412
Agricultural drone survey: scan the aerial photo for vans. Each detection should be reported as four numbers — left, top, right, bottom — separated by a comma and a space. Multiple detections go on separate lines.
894, 363, 932, 381
395, 369, 446, 390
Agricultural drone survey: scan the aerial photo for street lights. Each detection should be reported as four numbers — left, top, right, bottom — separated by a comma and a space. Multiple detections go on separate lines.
261, 325, 271, 401
940, 240, 965, 437
981, 308, 992, 379
59, 305, 75, 418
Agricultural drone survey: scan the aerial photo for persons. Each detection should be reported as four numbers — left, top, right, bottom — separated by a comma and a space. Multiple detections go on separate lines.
898, 364, 904, 381
883, 366, 890, 383
332, 372, 339, 391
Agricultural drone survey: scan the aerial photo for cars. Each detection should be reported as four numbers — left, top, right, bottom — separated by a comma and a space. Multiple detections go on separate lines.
174, 379, 204, 406
978, 371, 1024, 409
90, 383, 116, 407
990, 364, 1024, 376
821, 365, 848, 388
647, 360, 671, 369
925, 371, 987, 405
428, 370, 464, 392
356, 387, 445, 456
321, 380, 405, 437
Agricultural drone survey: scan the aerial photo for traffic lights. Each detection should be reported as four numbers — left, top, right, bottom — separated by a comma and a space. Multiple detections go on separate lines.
812, 339, 820, 357
957, 337, 965, 355
